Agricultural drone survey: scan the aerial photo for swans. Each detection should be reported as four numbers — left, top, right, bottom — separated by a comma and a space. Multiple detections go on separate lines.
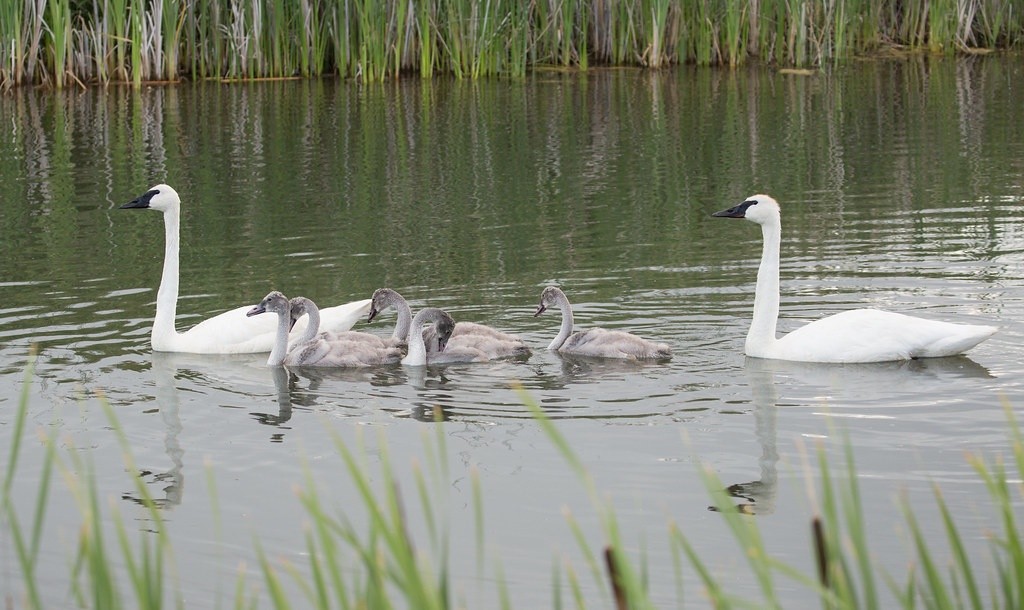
245, 286, 672, 370
114, 184, 375, 355
709, 189, 999, 363
